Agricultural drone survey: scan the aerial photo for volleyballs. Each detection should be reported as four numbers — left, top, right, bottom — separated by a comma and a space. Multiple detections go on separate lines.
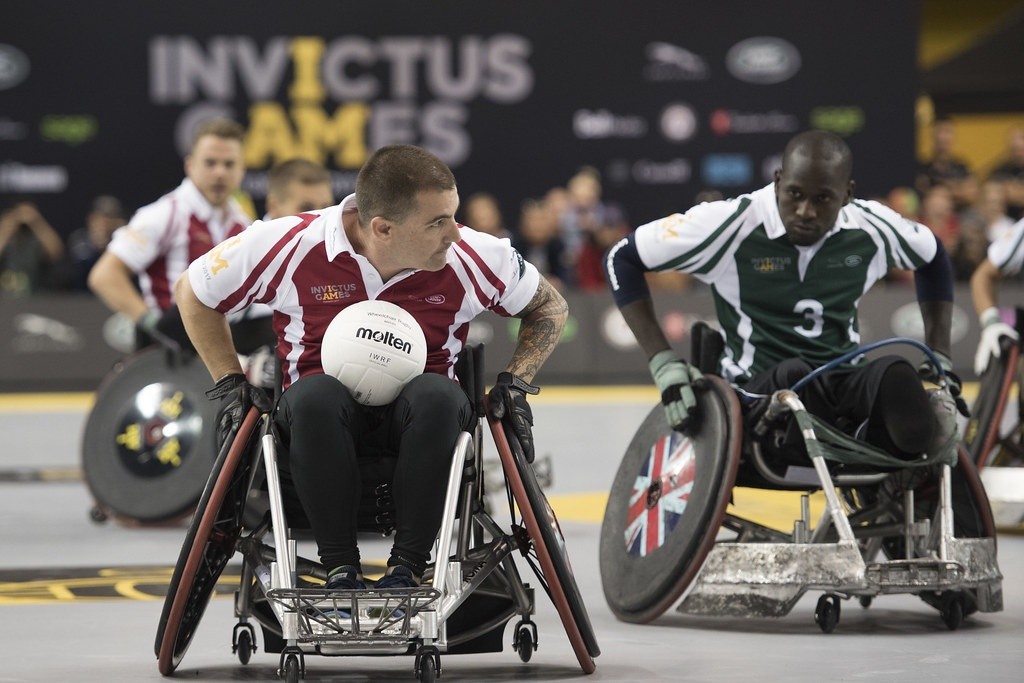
314, 297, 429, 411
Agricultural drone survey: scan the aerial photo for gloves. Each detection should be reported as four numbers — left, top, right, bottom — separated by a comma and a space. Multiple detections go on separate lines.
488, 372, 540, 462
972, 307, 1019, 377
920, 349, 970, 416
647, 347, 706, 431
204, 372, 272, 457
139, 310, 187, 366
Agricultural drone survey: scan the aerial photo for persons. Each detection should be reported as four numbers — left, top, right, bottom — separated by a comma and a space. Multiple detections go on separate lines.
604, 129, 971, 460
86, 118, 253, 366
263, 156, 340, 221
2, 107, 1024, 289
173, 143, 571, 590
964, 215, 1024, 376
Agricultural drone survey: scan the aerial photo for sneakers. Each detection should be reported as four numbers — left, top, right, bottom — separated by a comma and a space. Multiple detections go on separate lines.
315, 564, 364, 622
367, 563, 418, 617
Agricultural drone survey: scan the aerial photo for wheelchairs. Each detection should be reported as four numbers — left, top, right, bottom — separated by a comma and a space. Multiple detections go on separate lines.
78, 311, 221, 522
154, 341, 603, 683
595, 323, 1006, 633
961, 298, 1023, 467
